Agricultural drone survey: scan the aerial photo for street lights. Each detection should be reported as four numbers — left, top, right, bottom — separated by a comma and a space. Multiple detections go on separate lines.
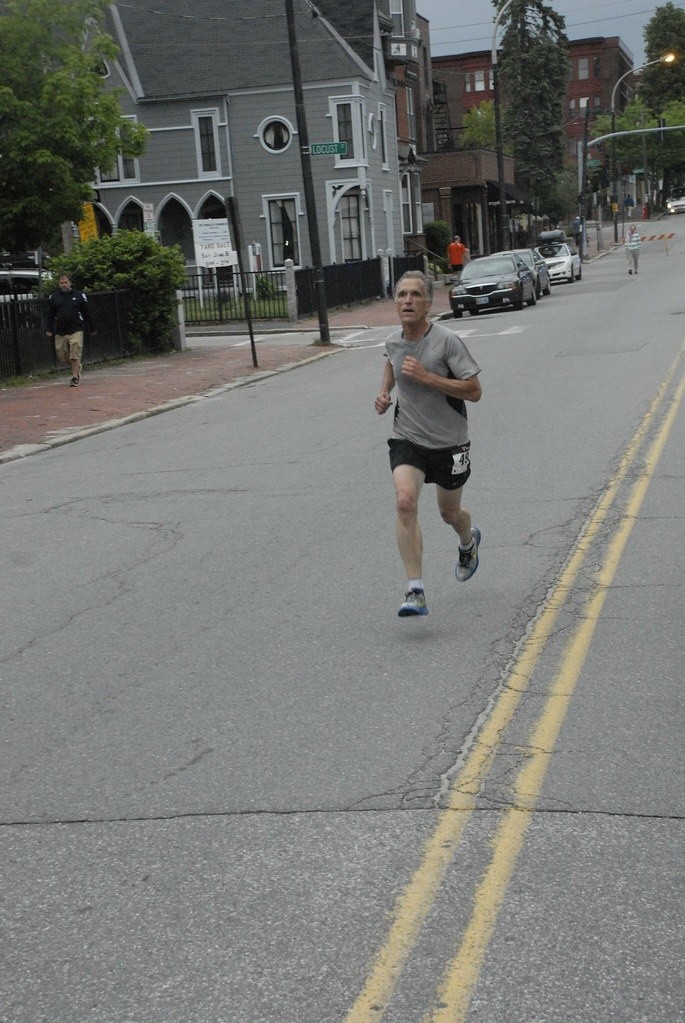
610, 53, 675, 241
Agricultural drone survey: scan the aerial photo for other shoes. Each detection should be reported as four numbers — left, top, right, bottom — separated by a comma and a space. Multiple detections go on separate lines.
629, 269, 638, 275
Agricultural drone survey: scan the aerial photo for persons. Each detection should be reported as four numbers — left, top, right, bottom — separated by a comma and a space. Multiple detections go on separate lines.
516, 226, 529, 249
374, 270, 482, 617
573, 216, 582, 246
45, 273, 98, 386
448, 235, 470, 285
624, 194, 634, 220
624, 225, 642, 275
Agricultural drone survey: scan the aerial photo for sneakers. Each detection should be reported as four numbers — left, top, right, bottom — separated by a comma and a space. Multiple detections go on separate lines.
455, 526, 481, 582
71, 364, 82, 386
397, 587, 429, 616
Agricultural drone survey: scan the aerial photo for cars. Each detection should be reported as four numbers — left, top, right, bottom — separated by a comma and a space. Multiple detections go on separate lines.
534, 239, 582, 284
1, 267, 56, 329
509, 248, 551, 300
666, 187, 685, 214
449, 250, 537, 318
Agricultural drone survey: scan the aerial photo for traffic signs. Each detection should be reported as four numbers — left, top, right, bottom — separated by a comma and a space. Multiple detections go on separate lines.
311, 142, 349, 156
